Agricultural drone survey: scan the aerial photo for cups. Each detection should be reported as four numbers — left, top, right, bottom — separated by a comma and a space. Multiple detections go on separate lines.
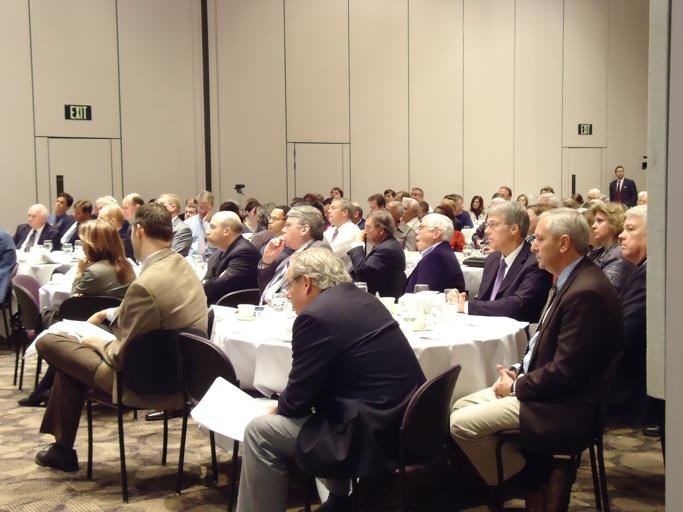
237, 304, 256, 317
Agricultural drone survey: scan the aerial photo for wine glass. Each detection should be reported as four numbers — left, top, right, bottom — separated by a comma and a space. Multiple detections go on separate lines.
414, 284, 429, 294
62, 243, 73, 257
353, 282, 368, 292
75, 240, 83, 253
273, 293, 287, 311
43, 240, 53, 252
444, 289, 460, 305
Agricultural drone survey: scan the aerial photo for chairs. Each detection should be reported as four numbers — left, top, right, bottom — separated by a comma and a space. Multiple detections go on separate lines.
305, 364, 462, 512
87, 328, 209, 503
51, 264, 70, 282
0, 264, 19, 347
12, 274, 44, 390
208, 289, 261, 337
176, 332, 279, 498
495, 351, 625, 512
35, 295, 124, 405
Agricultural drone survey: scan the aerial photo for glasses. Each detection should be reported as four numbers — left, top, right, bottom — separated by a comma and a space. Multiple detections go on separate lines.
267, 215, 286, 221
280, 275, 304, 297
485, 222, 513, 229
417, 223, 439, 231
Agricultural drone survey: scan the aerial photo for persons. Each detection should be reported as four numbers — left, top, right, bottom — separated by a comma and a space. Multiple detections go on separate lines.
36, 202, 208, 472
1, 166, 667, 424
235, 246, 428, 512
451, 207, 632, 499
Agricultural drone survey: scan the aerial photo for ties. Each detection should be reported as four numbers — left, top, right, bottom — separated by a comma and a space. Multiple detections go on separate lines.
489, 258, 508, 301
330, 227, 338, 244
616, 182, 621, 204
518, 286, 556, 375
24, 229, 37, 253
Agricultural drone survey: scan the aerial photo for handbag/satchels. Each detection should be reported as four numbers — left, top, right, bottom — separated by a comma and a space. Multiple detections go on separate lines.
462, 256, 487, 267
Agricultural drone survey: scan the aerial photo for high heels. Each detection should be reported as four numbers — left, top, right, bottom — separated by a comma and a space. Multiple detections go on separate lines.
18, 389, 50, 406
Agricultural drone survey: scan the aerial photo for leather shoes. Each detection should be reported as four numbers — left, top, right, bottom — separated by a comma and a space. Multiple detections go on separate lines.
35, 442, 79, 472
145, 409, 184, 422
546, 465, 576, 512
524, 490, 544, 512
